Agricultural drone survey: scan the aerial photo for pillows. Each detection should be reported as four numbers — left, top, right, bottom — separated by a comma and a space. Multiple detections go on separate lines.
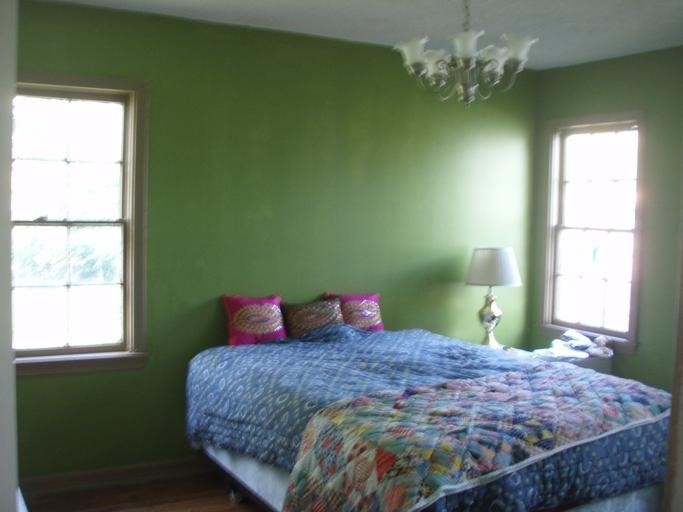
221, 291, 385, 346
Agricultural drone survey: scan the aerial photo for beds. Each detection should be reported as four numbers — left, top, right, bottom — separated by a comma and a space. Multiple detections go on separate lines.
184, 329, 672, 512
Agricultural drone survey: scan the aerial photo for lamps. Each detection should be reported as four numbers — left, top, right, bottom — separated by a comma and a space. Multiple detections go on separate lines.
463, 246, 523, 348
389, 1, 540, 111
